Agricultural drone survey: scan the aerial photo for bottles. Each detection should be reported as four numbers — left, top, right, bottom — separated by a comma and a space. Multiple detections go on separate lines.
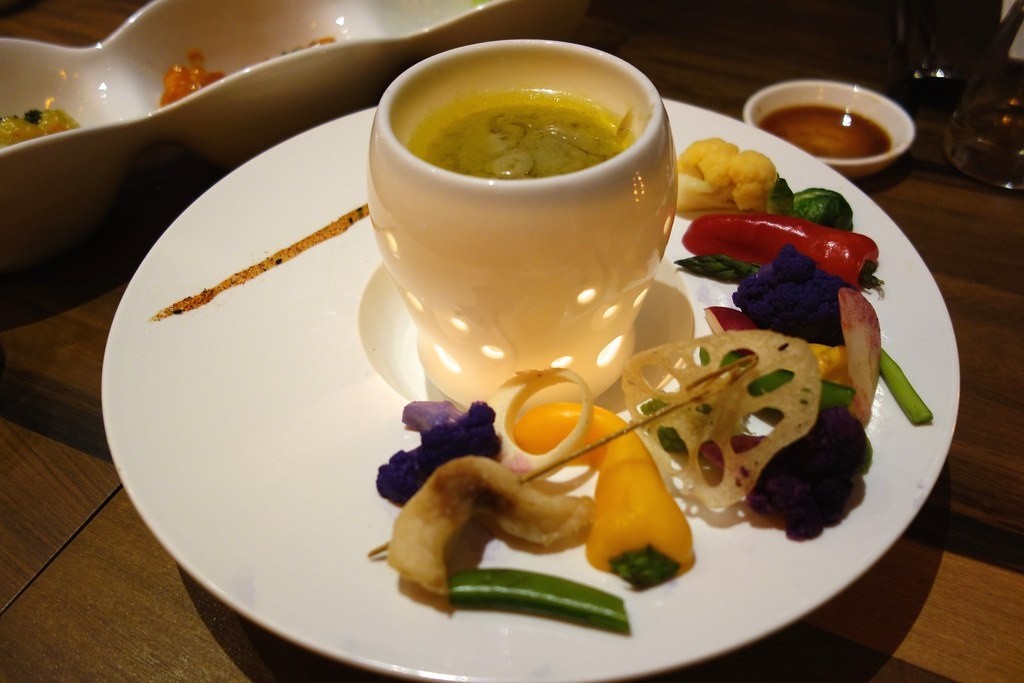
943, 0, 1024, 191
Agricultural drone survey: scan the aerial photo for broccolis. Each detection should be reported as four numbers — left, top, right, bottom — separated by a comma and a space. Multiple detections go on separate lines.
731, 245, 862, 352
676, 138, 779, 214
700, 402, 868, 544
378, 401, 505, 505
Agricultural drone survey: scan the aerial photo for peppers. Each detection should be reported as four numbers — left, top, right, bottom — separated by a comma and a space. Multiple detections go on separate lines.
681, 211, 888, 294
512, 400, 696, 590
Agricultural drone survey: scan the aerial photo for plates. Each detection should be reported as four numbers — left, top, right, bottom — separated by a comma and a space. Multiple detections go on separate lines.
742, 79, 916, 179
100, 94, 965, 683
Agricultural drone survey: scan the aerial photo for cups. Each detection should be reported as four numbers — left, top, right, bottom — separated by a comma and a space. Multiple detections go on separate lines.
369, 38, 678, 418
